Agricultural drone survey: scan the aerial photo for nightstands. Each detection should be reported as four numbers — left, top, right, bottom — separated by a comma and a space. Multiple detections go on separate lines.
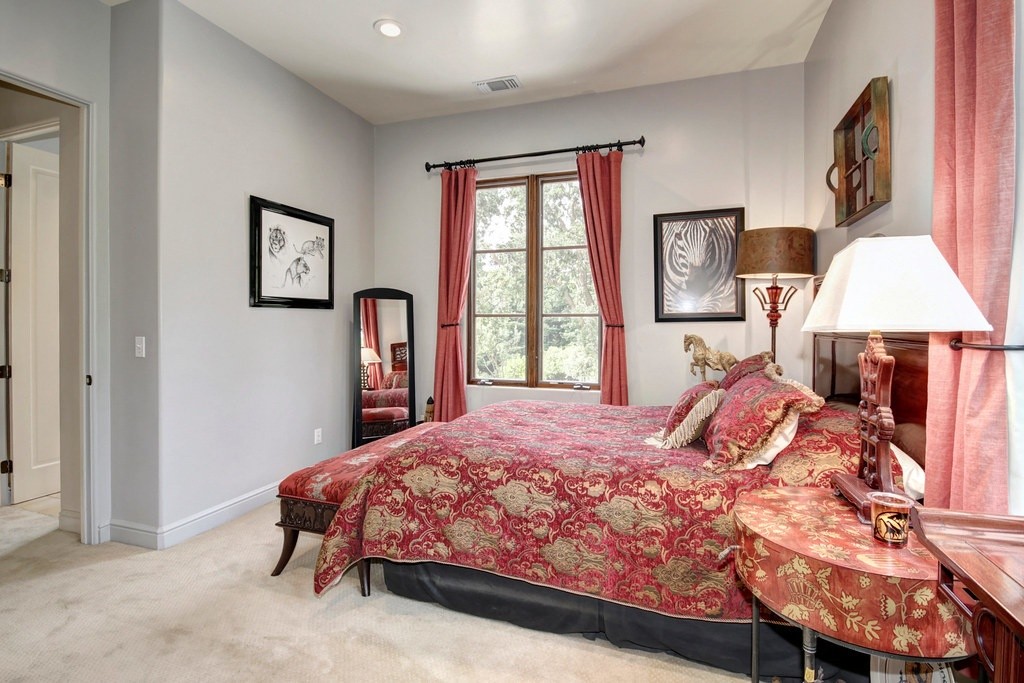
732, 486, 982, 682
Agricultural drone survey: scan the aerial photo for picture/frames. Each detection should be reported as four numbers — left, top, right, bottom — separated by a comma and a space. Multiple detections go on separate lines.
249, 194, 334, 309
652, 206, 746, 322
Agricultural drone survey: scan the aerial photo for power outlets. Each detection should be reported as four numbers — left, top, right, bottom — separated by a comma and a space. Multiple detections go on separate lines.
314, 427, 322, 444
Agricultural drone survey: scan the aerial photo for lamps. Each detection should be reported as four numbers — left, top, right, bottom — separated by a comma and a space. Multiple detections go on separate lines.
360, 347, 382, 389
734, 226, 817, 364
801, 234, 993, 523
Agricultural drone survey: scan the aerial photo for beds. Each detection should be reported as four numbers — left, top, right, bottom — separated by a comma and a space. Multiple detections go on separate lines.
314, 328, 929, 683
361, 342, 409, 444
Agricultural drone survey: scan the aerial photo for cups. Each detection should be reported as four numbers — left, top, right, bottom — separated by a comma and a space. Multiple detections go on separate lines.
865, 491, 915, 549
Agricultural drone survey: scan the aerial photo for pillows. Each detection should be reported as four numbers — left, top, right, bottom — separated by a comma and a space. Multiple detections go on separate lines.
700, 351, 774, 439
642, 379, 726, 449
392, 374, 408, 388
383, 371, 405, 388
701, 363, 825, 473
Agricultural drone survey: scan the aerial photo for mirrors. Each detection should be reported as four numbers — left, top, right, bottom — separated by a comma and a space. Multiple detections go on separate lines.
351, 287, 415, 449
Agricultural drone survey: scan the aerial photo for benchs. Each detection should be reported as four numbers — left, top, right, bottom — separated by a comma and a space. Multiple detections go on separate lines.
361, 407, 409, 440
271, 420, 457, 598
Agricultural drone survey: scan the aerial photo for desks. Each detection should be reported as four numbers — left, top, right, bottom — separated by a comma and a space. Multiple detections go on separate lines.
910, 506, 1024, 682
